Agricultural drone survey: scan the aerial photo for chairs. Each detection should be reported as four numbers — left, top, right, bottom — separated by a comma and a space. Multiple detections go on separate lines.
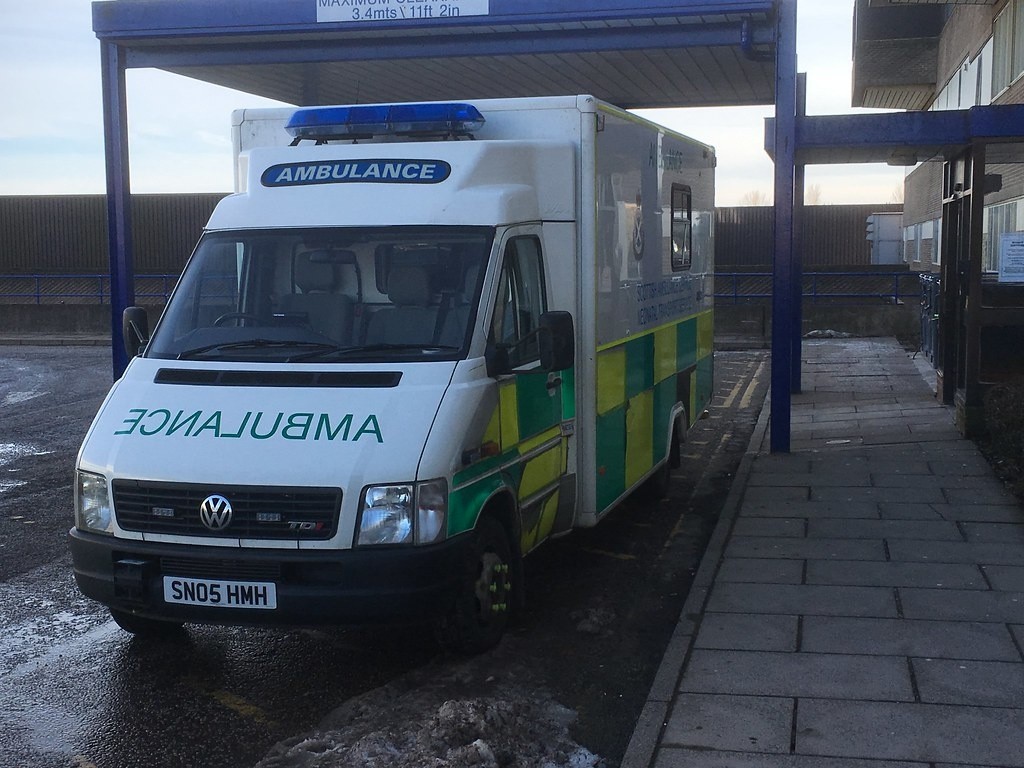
275, 250, 362, 348
362, 264, 463, 349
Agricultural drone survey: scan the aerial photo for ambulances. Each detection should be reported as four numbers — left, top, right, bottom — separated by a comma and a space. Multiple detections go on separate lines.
67, 92, 718, 649
